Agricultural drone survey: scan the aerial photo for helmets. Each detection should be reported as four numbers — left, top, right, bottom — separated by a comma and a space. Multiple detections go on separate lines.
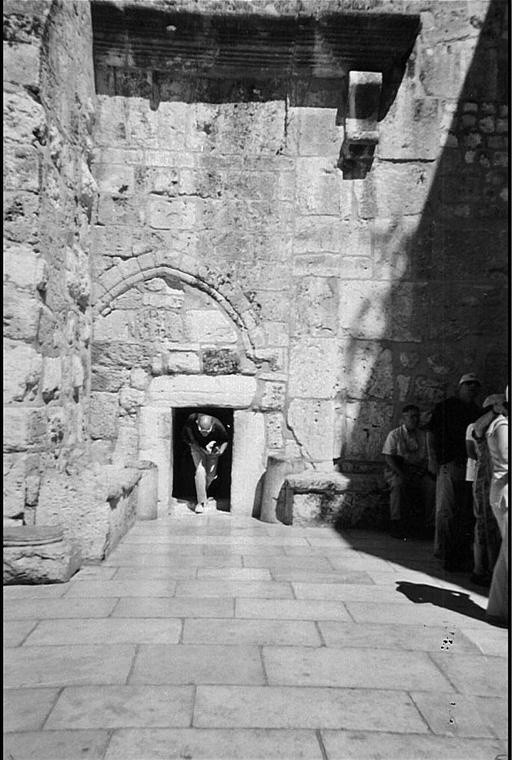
197, 415, 213, 433
459, 372, 484, 388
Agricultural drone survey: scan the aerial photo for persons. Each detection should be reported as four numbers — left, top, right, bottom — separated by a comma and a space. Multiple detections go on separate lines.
381, 371, 510, 631
182, 412, 229, 515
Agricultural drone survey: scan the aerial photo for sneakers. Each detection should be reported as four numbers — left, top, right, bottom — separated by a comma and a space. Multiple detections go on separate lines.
470, 570, 491, 587
392, 521, 406, 539
484, 614, 508, 628
444, 557, 463, 573
195, 502, 206, 513
